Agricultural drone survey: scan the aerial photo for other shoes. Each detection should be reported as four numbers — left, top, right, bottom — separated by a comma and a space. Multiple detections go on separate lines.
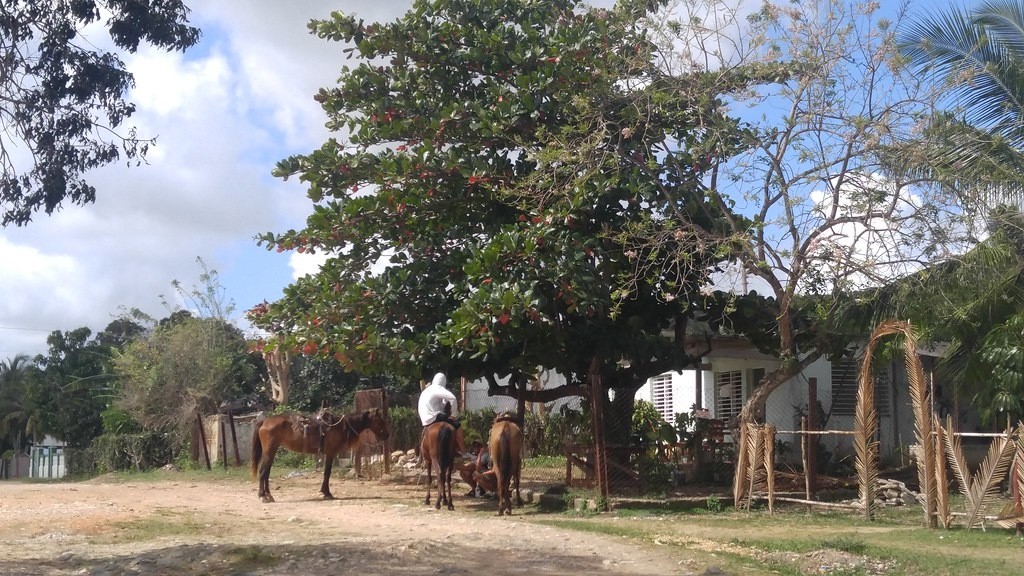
480, 493, 492, 499
465, 492, 474, 497
491, 495, 499, 500
462, 452, 477, 459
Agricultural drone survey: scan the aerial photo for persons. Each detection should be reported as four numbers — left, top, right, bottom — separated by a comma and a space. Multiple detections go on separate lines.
458, 439, 499, 499
418, 373, 478, 460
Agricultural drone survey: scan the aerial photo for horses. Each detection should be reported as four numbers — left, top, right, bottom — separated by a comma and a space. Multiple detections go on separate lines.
420, 420, 457, 511
251, 406, 390, 503
489, 410, 524, 517
